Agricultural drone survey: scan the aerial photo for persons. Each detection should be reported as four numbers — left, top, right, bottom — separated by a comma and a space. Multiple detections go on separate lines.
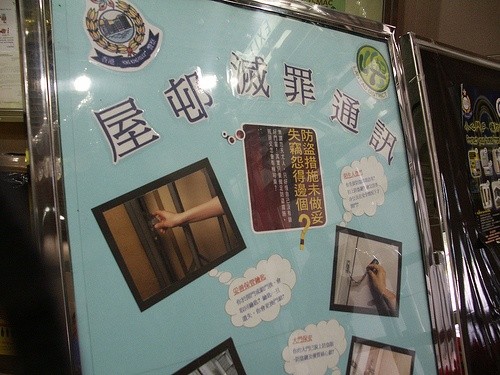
366, 264, 396, 313
150, 194, 225, 236
